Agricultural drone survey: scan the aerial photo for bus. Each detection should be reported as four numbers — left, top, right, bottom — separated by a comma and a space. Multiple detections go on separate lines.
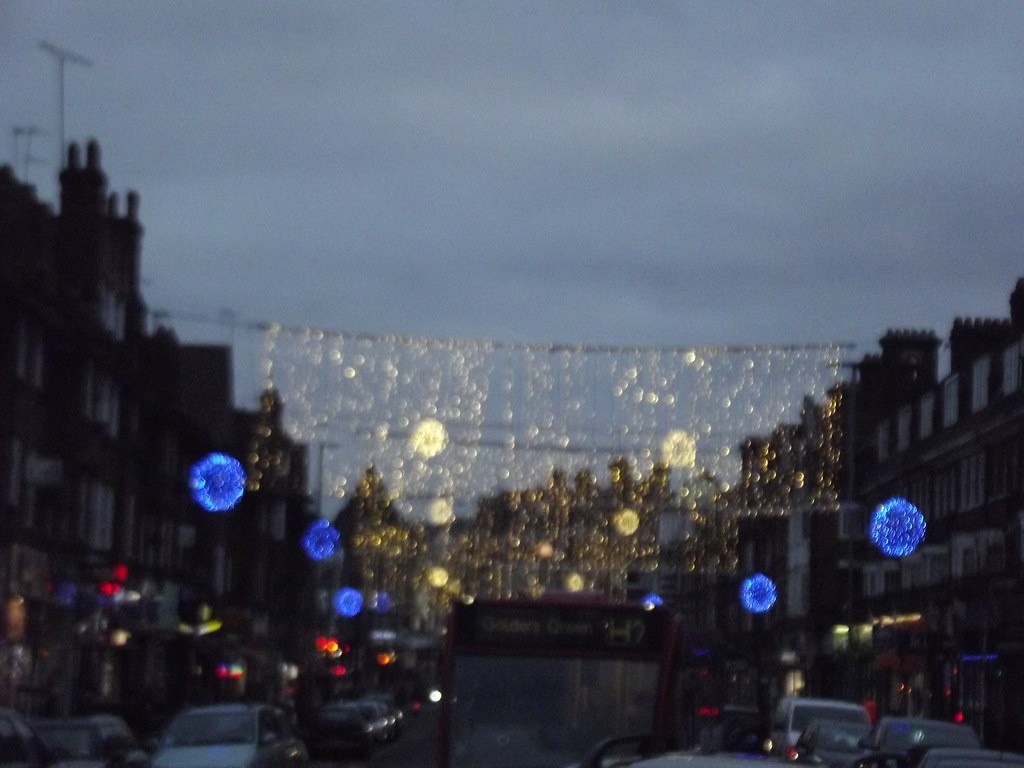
436, 593, 685, 768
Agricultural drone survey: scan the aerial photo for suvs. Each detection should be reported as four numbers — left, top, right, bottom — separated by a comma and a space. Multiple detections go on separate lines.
858, 717, 979, 765
152, 705, 304, 768
792, 720, 872, 768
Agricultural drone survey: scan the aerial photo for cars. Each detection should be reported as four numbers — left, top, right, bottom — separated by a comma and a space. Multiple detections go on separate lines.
28, 716, 148, 768
914, 748, 1024, 768
0, 708, 57, 768
307, 698, 404, 752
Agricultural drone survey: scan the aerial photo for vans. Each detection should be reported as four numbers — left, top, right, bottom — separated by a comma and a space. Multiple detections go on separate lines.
768, 698, 871, 762
